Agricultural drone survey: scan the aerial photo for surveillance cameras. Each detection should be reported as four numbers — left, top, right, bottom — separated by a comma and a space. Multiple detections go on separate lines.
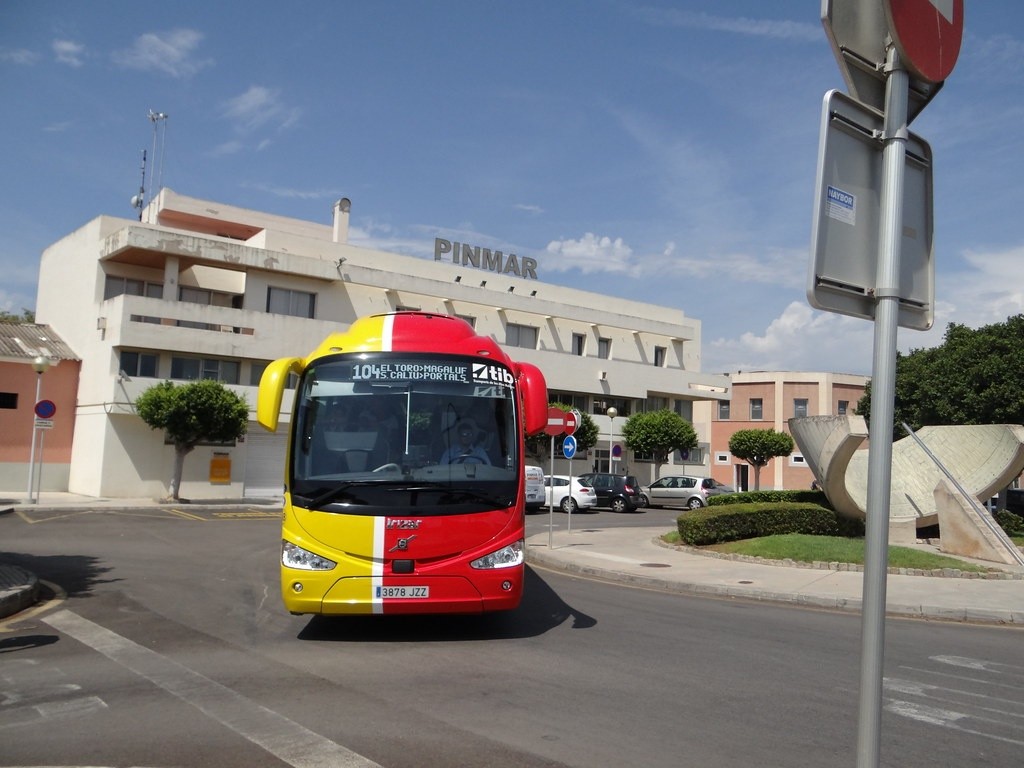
120, 369, 132, 383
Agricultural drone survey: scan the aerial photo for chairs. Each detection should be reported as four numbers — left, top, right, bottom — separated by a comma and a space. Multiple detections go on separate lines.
670, 480, 678, 488
680, 480, 686, 487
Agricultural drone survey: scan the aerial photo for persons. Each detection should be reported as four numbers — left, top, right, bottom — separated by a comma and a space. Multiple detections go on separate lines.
441, 424, 492, 467
318, 394, 401, 461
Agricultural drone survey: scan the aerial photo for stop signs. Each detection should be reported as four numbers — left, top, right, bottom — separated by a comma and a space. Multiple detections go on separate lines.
543, 408, 566, 436
565, 413, 577, 436
890, 0, 964, 82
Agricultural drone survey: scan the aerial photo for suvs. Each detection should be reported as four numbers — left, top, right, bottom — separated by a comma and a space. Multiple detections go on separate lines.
523, 466, 545, 514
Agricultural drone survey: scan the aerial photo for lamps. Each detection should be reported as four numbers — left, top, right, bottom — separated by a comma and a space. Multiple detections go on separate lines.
455, 276, 461, 284
507, 285, 515, 294
480, 280, 487, 288
529, 290, 537, 297
118, 369, 132, 385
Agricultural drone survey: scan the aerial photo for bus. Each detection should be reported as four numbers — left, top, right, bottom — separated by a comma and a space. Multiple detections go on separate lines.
253, 312, 548, 617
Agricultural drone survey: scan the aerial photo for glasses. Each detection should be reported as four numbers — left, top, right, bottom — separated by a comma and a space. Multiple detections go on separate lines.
459, 432, 473, 437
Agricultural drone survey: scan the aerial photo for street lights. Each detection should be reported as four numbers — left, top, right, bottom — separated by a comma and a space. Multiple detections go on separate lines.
26, 355, 49, 499
607, 407, 617, 473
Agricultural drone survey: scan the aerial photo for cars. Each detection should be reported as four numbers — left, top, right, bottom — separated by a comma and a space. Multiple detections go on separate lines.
680, 478, 734, 494
639, 474, 718, 511
543, 474, 597, 513
579, 473, 646, 512
